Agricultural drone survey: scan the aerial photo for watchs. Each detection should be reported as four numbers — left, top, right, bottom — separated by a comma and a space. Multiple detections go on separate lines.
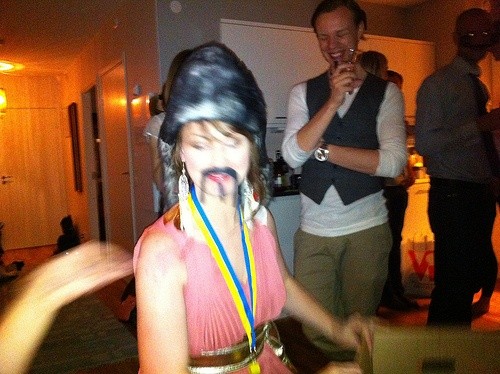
314, 143, 329, 162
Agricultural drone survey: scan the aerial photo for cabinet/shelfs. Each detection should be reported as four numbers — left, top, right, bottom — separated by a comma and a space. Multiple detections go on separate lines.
218, 18, 436, 128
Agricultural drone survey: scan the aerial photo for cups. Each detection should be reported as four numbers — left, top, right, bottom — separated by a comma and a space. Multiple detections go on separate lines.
342, 48, 365, 73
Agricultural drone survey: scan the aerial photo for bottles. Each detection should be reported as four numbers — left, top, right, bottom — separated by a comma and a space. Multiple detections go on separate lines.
266, 150, 305, 190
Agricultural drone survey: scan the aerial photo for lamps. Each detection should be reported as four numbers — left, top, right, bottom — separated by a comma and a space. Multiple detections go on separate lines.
0, 88, 6, 119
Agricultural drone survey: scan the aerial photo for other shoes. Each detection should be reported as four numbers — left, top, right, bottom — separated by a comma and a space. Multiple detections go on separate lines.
380, 297, 419, 311
472, 300, 489, 313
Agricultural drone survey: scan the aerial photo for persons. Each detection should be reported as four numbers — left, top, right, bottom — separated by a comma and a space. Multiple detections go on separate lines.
132, 42, 384, 374
279, 0, 406, 353
356, 50, 420, 313
414, 7, 500, 329
0, 240, 134, 374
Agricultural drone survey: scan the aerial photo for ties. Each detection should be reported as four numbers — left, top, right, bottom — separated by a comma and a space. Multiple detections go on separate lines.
471, 76, 500, 178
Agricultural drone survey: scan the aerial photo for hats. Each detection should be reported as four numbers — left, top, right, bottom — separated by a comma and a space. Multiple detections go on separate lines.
160, 39, 266, 146
